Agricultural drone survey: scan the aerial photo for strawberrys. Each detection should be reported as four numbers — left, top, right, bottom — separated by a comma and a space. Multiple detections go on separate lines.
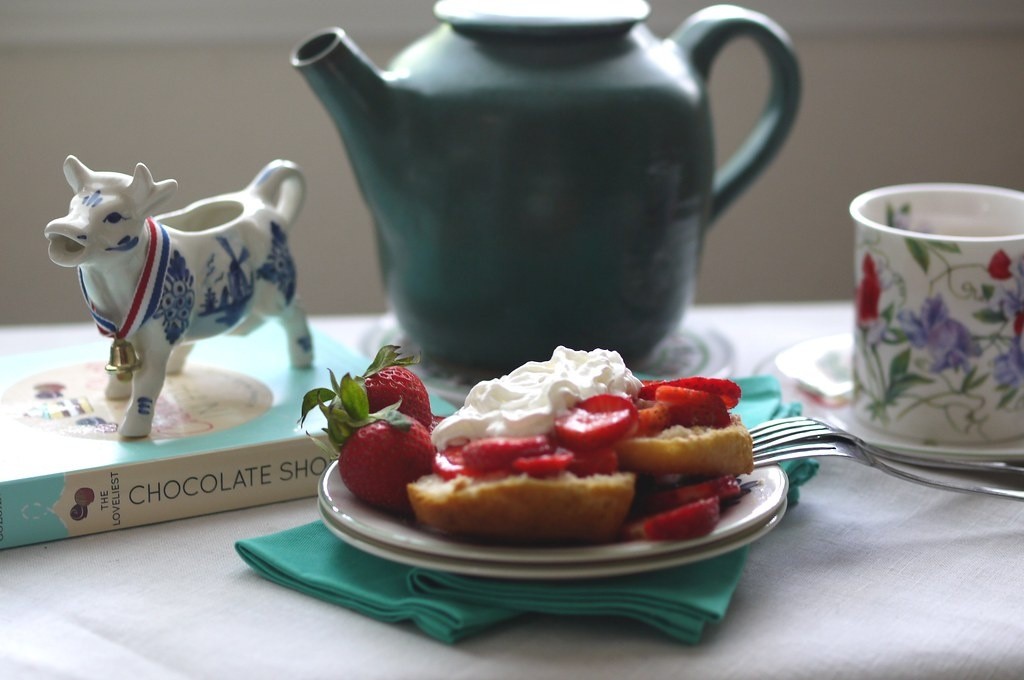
291, 342, 743, 541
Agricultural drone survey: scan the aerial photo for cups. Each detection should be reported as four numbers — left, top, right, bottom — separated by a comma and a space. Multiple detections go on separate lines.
846, 181, 1024, 445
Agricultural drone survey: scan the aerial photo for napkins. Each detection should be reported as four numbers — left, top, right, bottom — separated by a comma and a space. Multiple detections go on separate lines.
235, 376, 819, 649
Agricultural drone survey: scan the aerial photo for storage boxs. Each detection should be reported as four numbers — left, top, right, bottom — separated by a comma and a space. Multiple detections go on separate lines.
0, 321, 460, 550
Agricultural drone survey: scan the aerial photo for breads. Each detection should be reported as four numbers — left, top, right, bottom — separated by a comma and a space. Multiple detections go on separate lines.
614, 406, 754, 473
406, 461, 636, 541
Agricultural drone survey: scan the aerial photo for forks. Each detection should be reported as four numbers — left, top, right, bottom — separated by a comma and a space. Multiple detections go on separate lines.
745, 414, 1024, 500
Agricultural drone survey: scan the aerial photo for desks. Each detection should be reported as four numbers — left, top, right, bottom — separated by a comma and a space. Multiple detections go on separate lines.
0, 299, 1024, 680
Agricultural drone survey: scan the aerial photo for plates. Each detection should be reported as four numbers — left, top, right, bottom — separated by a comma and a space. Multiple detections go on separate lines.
314, 456, 790, 581
353, 313, 739, 409
775, 332, 1024, 460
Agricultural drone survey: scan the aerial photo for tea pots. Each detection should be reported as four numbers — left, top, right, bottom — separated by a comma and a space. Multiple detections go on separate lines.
286, 0, 805, 380
